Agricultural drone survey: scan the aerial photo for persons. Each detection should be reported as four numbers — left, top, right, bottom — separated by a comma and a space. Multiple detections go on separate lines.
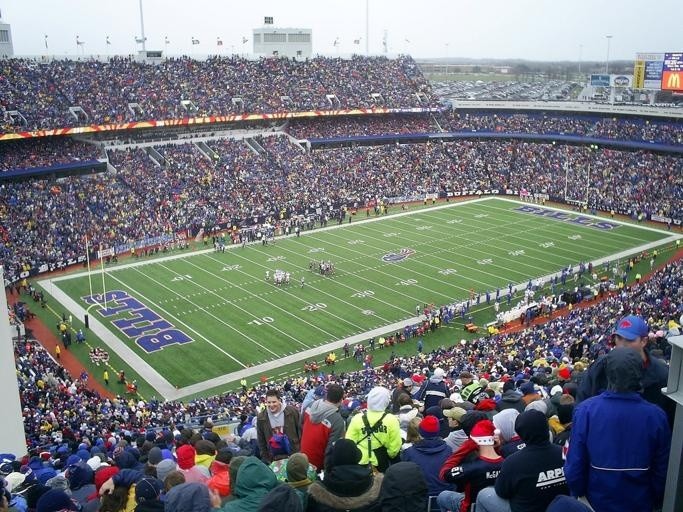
1, 55, 683, 512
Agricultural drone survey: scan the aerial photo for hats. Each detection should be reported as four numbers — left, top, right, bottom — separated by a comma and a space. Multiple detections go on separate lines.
418, 415, 439, 439
443, 407, 468, 421
135, 478, 159, 501
328, 438, 362, 467
612, 315, 647, 339
469, 419, 496, 447
403, 378, 414, 386
